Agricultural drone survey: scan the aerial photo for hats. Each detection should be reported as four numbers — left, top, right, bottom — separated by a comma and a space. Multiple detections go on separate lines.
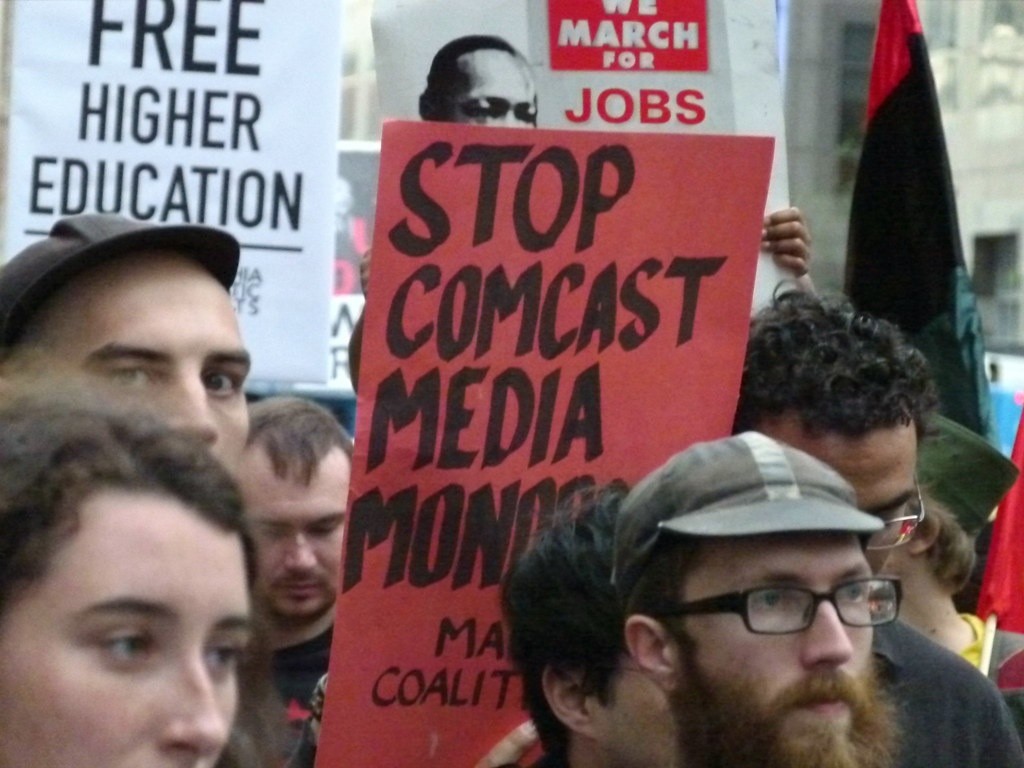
613, 430, 886, 594
915, 409, 1019, 540
2, 215, 240, 356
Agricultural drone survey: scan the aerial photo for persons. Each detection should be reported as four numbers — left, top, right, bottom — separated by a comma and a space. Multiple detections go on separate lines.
0, 398, 256, 768
0, 215, 251, 466
348, 206, 1024, 768
234, 397, 354, 768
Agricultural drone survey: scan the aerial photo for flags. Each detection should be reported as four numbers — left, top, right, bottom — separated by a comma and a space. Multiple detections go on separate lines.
976, 409, 1024, 691
846, 0, 993, 443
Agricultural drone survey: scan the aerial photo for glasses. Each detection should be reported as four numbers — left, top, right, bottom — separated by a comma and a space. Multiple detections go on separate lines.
662, 577, 901, 635
437, 96, 538, 125
863, 480, 925, 549
